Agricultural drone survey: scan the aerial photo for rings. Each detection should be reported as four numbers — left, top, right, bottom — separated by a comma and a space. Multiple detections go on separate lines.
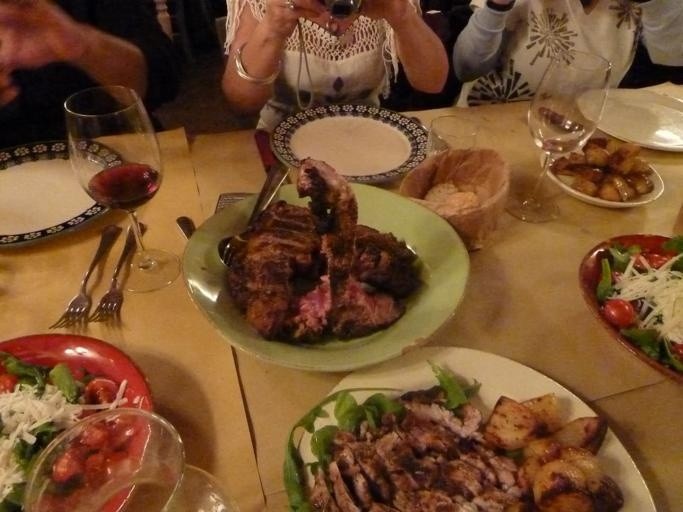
285, 2, 294, 11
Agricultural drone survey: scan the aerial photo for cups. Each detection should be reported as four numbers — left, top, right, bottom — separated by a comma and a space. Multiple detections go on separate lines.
428, 115, 480, 156
22, 409, 242, 512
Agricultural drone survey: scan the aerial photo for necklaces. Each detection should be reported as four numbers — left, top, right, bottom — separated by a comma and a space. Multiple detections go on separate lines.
326, 15, 339, 33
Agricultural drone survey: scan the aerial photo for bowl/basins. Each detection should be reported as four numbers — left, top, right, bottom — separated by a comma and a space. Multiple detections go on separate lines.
401, 148, 510, 244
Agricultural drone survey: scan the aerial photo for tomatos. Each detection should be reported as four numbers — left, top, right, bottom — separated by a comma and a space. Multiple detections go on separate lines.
600, 295, 634, 332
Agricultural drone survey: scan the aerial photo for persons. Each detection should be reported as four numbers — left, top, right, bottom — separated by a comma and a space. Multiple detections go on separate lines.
1, 0, 181, 146
219, 1, 449, 130
454, 0, 682, 106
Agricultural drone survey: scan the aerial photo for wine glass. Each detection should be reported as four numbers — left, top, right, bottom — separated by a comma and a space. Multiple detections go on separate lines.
506, 49, 612, 223
64, 84, 182, 294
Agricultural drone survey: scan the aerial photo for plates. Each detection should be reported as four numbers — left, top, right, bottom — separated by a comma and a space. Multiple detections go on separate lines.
269, 105, 428, 184
298, 346, 657, 512
541, 155, 666, 210
576, 88, 683, 153
181, 182, 470, 372
578, 234, 682, 386
0, 137, 123, 249
0, 334, 151, 512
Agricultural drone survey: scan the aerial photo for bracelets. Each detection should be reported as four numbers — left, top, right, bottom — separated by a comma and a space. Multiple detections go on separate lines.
235, 41, 283, 86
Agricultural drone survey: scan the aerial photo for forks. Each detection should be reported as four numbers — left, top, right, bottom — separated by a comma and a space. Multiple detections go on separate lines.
48, 225, 122, 329
87, 221, 146, 323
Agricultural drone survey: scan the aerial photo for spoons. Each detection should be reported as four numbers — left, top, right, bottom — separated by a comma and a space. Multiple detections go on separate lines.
217, 164, 290, 267
